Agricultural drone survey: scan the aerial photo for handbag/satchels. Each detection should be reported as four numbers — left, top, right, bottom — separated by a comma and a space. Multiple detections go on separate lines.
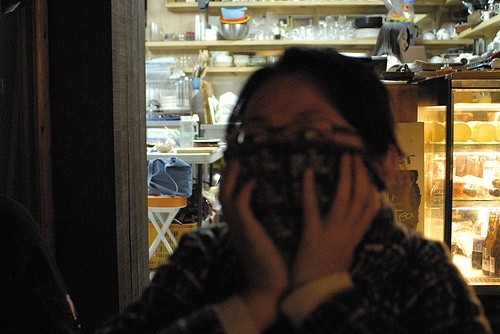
148, 157, 192, 198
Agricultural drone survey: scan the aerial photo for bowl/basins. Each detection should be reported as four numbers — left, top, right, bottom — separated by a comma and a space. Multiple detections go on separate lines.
209, 50, 279, 67
156, 142, 173, 153
218, 6, 251, 40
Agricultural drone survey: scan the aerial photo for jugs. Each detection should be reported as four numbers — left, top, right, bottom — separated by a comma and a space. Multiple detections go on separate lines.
178, 119, 199, 149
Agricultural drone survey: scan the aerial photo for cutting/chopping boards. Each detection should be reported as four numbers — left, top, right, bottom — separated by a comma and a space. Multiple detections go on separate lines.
177, 147, 219, 154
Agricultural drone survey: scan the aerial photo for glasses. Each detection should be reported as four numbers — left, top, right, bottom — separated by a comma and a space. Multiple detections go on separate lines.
223, 117, 361, 145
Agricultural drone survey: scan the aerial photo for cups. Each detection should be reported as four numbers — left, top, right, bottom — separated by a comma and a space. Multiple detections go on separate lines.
481, 11, 494, 21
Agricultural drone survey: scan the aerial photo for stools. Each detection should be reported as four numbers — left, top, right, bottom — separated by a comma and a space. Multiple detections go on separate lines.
148, 196, 186, 261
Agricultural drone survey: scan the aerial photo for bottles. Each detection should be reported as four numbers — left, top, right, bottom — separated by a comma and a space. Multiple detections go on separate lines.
481, 212, 497, 276
189, 77, 206, 140
472, 213, 488, 269
489, 212, 500, 278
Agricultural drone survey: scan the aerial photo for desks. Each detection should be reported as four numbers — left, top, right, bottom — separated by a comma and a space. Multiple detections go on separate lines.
148, 147, 224, 231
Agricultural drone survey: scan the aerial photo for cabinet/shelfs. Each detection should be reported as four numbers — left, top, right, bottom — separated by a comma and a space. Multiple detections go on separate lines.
146, 0, 500, 298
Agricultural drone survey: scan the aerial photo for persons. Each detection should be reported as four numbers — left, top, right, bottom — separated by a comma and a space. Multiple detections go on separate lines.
374, 21, 411, 70
88, 46, 491, 333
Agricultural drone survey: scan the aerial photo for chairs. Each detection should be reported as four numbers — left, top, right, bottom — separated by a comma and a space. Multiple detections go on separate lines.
0, 196, 80, 334
